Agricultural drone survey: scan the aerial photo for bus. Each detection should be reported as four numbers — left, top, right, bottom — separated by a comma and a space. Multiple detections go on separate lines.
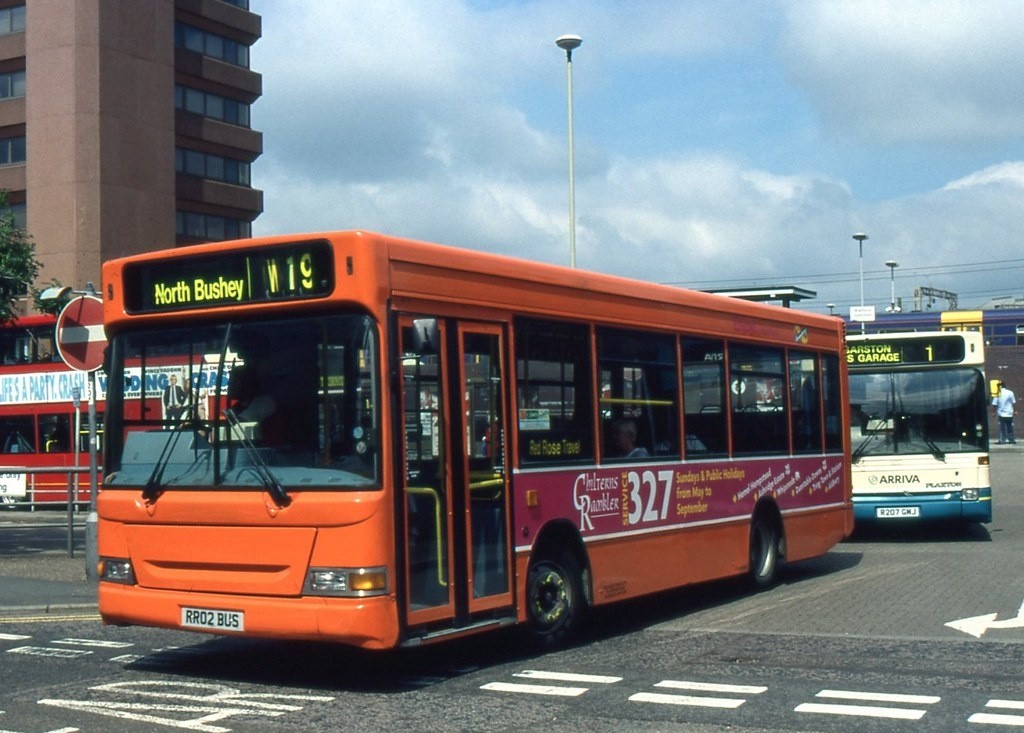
97, 232, 853, 649
0, 315, 246, 505
801, 327, 995, 525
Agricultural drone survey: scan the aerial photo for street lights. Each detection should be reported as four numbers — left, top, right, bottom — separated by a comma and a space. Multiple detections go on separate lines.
826, 304, 837, 317
556, 34, 584, 269
884, 260, 898, 312
853, 230, 870, 335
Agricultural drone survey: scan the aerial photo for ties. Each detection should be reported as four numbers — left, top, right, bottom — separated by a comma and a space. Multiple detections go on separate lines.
172, 385, 175, 406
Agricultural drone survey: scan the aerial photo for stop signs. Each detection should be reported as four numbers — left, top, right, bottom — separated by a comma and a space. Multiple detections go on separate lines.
55, 295, 108, 371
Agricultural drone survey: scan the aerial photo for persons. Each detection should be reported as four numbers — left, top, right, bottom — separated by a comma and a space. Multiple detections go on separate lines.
991, 382, 1018, 444
164, 374, 185, 429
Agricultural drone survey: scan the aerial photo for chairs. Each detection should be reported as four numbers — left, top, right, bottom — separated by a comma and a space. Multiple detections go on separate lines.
3, 352, 51, 365
602, 403, 813, 451
